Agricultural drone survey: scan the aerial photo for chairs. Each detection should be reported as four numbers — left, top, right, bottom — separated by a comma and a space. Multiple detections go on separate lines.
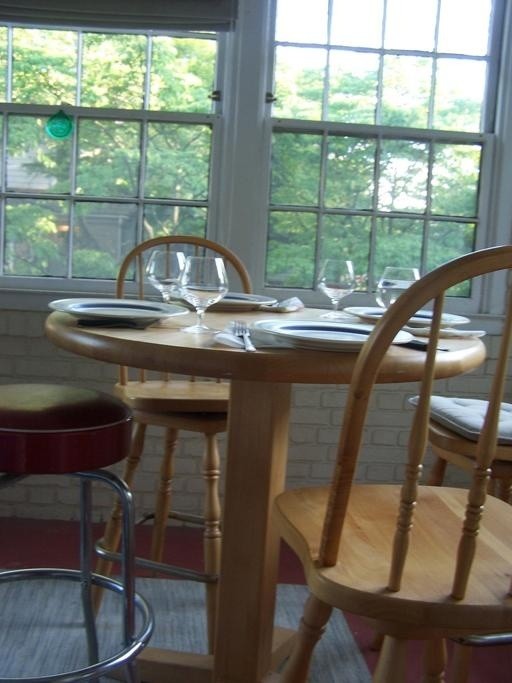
81, 235, 254, 655
272, 246, 512, 683
406, 393, 512, 683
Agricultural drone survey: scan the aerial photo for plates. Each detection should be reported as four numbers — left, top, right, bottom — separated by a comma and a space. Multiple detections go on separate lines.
48, 298, 191, 327
208, 292, 278, 312
255, 319, 414, 352
342, 307, 472, 331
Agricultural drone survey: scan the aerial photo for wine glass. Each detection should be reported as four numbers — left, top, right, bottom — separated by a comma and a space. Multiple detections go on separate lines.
146, 250, 228, 334
316, 260, 355, 319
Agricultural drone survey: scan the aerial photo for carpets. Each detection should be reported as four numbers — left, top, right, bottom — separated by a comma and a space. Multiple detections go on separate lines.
1, 573, 371, 683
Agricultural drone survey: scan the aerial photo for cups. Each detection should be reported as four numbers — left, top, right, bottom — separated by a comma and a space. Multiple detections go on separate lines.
375, 266, 421, 330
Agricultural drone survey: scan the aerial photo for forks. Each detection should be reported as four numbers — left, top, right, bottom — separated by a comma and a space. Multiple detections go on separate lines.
233, 320, 257, 352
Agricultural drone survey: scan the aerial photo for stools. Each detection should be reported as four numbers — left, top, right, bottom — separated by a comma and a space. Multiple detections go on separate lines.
2, 382, 156, 683
